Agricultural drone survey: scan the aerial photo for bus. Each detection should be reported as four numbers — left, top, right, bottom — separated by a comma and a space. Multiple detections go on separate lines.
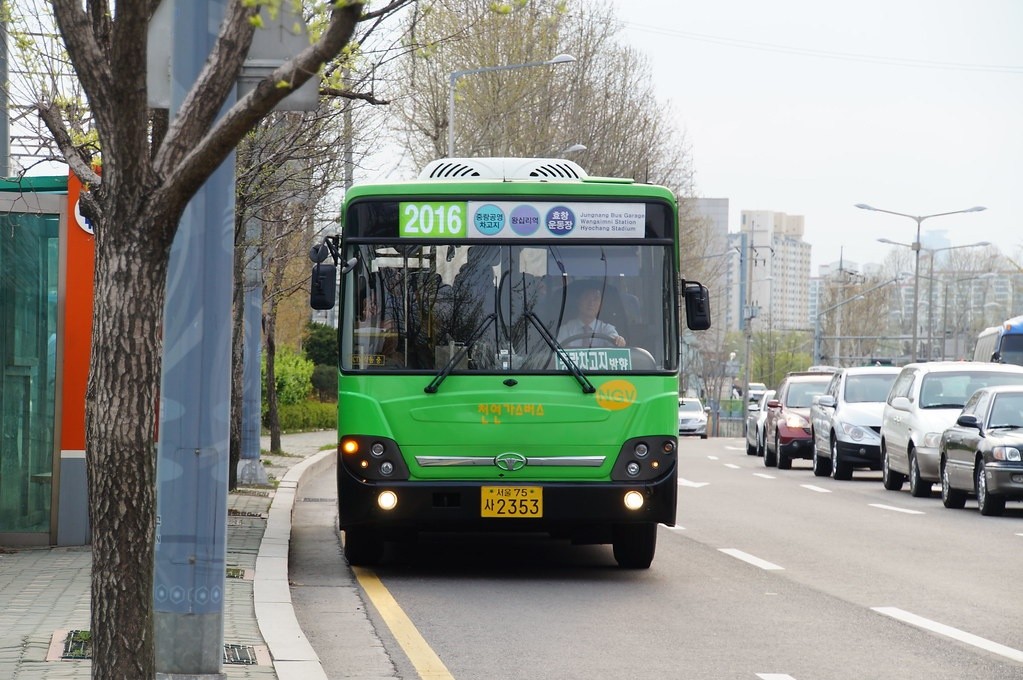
310, 155, 711, 572
972, 314, 1023, 367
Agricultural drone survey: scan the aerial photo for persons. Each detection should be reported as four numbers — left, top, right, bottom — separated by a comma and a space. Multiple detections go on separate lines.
551, 287, 626, 347
354, 320, 410, 369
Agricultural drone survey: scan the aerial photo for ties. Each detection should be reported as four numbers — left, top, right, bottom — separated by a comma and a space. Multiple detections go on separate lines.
583, 324, 592, 347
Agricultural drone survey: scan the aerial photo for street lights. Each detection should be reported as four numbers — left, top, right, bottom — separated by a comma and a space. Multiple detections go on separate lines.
448, 54, 577, 158
854, 203, 988, 364
875, 237, 992, 360
898, 270, 998, 361
549, 145, 589, 159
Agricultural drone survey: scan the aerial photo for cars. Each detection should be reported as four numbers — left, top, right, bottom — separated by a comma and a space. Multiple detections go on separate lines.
763, 370, 834, 469
937, 384, 1023, 516
809, 366, 903, 481
678, 396, 711, 440
749, 382, 768, 402
878, 361, 1023, 498
745, 389, 777, 456
